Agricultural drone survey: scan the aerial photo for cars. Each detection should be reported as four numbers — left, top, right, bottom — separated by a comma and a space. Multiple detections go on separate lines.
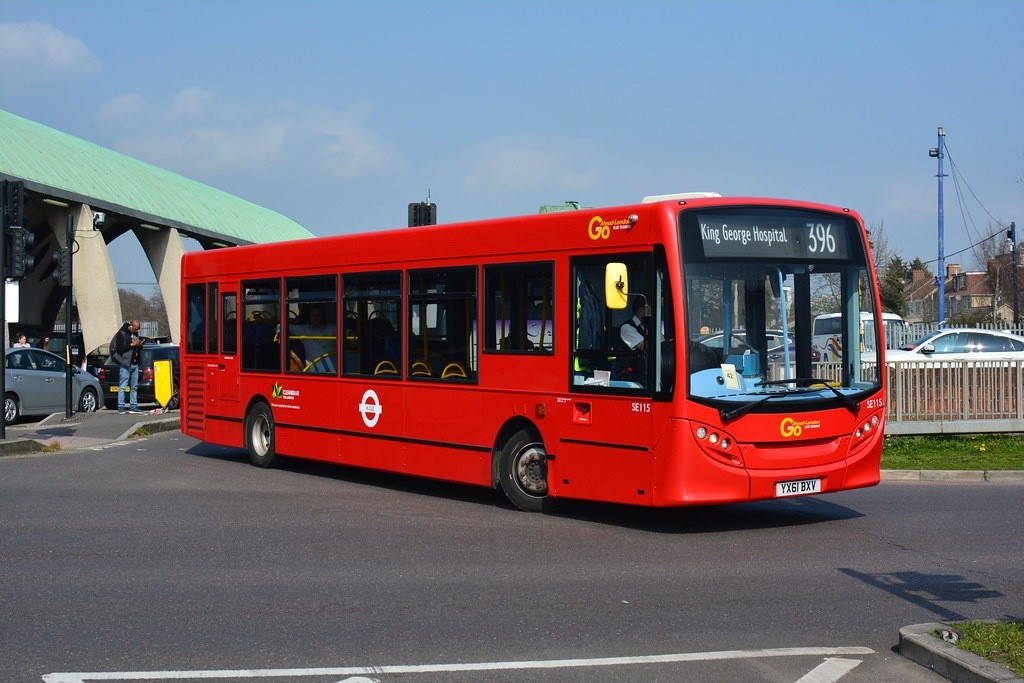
81, 342, 180, 410
890, 328, 1024, 365
5, 347, 105, 425
694, 328, 821, 369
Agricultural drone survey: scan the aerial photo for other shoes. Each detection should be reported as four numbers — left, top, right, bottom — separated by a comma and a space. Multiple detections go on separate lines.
117, 407, 126, 414
129, 406, 143, 413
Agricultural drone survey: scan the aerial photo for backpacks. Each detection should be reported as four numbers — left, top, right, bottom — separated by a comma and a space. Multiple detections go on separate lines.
110, 331, 127, 364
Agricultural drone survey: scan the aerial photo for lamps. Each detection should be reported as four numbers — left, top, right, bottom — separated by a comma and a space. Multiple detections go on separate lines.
92, 211, 106, 231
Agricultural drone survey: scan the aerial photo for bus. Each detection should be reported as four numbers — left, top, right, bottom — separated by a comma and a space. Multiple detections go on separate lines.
179, 192, 884, 513
811, 312, 908, 368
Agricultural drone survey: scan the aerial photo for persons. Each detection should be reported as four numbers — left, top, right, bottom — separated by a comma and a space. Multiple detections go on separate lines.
166, 335, 172, 342
12, 332, 32, 367
274, 305, 359, 374
618, 296, 652, 381
116, 319, 145, 414
34, 335, 52, 368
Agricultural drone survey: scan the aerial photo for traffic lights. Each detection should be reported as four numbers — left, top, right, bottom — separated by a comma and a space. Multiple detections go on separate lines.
52, 250, 72, 287
13, 231, 37, 277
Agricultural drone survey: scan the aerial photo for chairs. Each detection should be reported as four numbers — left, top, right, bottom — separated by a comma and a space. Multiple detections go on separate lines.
20, 355, 32, 369
194, 310, 468, 379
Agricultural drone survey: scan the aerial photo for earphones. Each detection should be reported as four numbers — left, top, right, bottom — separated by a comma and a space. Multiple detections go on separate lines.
130, 326, 132, 328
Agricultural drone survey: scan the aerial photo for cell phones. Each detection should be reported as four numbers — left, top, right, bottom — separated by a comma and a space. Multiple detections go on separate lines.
141, 339, 145, 344
